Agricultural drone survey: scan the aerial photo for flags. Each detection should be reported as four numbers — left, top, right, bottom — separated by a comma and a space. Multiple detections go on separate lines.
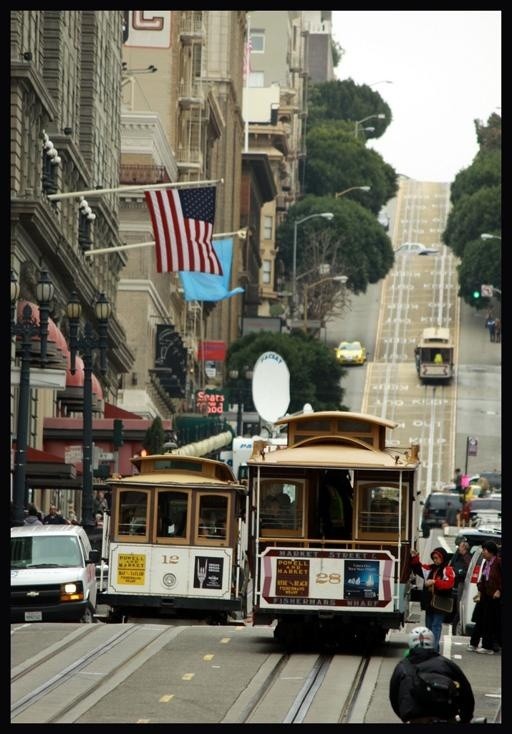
142, 187, 223, 277
179, 238, 243, 301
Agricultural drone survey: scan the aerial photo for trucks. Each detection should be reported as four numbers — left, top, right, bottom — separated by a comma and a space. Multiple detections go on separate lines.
228, 435, 296, 558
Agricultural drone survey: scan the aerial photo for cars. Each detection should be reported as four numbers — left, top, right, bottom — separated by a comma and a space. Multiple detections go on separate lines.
82, 529, 126, 625
394, 172, 415, 181
334, 339, 369, 366
392, 240, 439, 258
9, 524, 98, 625
478, 231, 501, 240
378, 209, 391, 230
419, 467, 500, 635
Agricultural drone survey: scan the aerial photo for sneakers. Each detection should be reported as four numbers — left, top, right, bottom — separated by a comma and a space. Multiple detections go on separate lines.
476, 648, 495, 655
467, 646, 477, 651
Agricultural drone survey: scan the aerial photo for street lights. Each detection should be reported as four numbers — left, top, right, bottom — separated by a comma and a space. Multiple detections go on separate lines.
287, 67, 392, 335
10, 265, 56, 522
64, 291, 110, 525
228, 360, 254, 436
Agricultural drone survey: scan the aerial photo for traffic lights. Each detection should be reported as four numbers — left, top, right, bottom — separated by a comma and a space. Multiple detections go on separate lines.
470, 281, 481, 300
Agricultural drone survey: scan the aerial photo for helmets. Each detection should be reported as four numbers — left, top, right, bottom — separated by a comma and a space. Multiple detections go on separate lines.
408, 627, 435, 649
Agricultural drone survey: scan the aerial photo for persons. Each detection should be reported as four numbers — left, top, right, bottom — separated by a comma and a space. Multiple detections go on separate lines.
23, 491, 109, 526
410, 468, 502, 653
263, 467, 398, 548
390, 626, 474, 723
199, 520, 227, 539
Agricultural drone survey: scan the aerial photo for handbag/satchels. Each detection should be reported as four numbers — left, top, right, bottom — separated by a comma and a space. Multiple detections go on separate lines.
410, 672, 459, 708
431, 596, 454, 613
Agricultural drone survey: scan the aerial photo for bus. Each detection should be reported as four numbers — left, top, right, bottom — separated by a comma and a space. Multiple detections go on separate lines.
103, 453, 246, 627
415, 326, 457, 385
244, 409, 424, 653
